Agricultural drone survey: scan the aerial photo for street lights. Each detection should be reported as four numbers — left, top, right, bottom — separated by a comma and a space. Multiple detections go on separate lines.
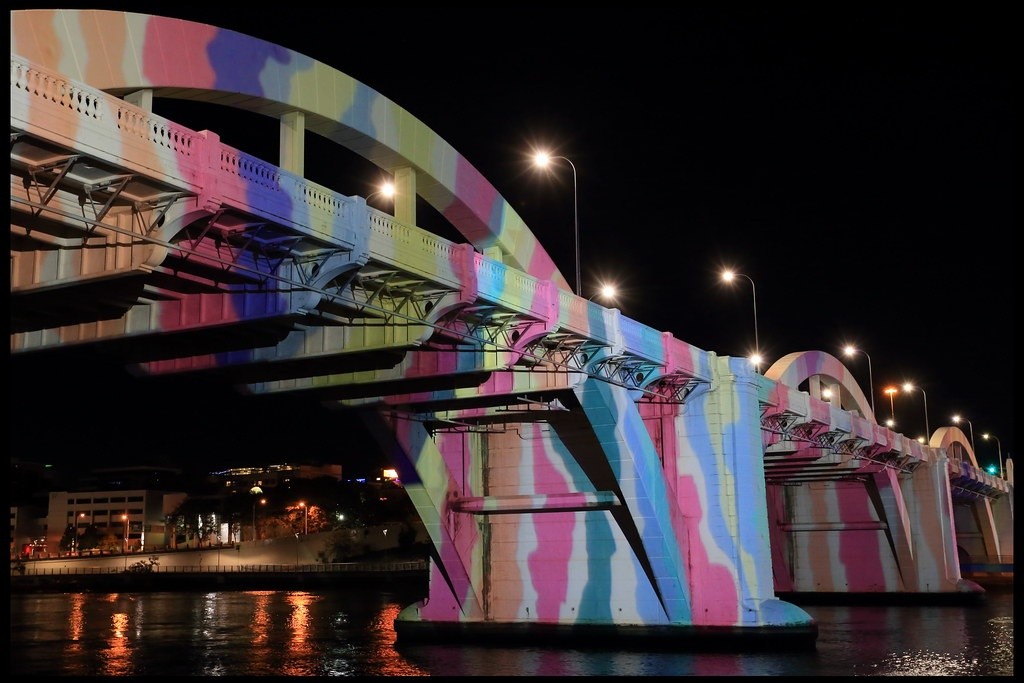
122, 516, 129, 539
537, 155, 581, 296
905, 385, 929, 447
724, 272, 760, 376
984, 434, 1003, 479
253, 500, 266, 541
846, 348, 874, 417
75, 513, 85, 551
885, 389, 897, 420
366, 187, 392, 202
954, 417, 974, 452
301, 503, 307, 535
589, 289, 612, 301
